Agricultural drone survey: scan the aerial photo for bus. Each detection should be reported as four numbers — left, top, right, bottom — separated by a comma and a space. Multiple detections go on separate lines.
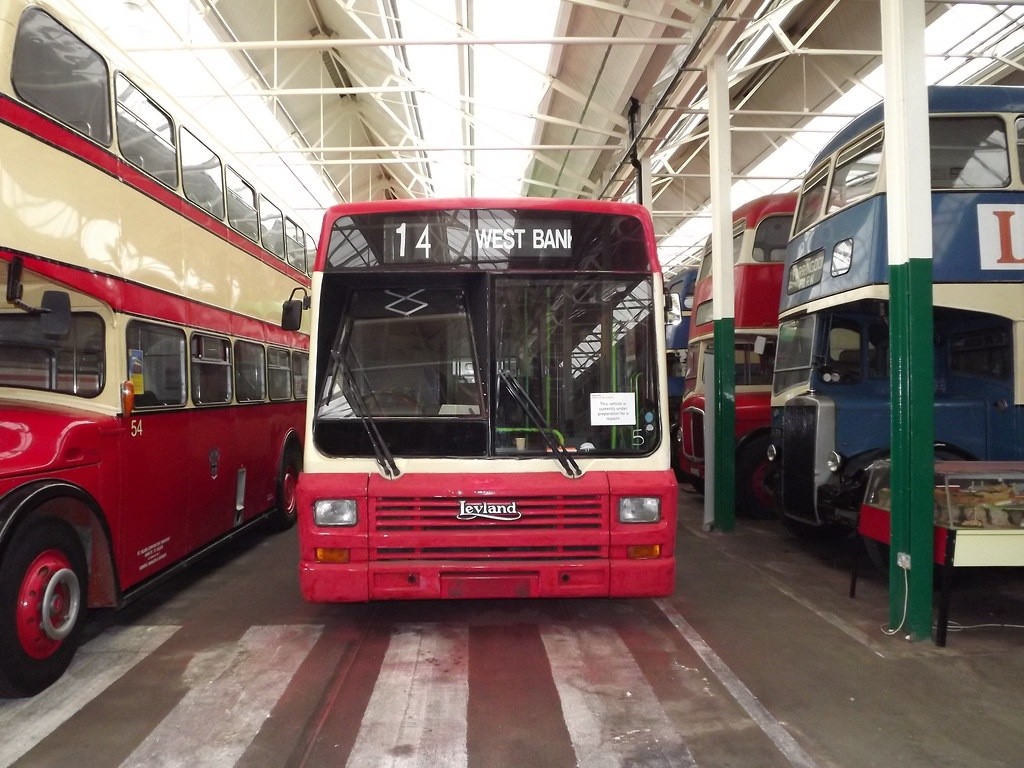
279, 196, 675, 602
0, 0, 313, 698
664, 82, 1024, 601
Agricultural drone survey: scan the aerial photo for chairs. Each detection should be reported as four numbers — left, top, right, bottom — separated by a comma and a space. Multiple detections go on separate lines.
364, 359, 432, 413
0, 340, 104, 398
